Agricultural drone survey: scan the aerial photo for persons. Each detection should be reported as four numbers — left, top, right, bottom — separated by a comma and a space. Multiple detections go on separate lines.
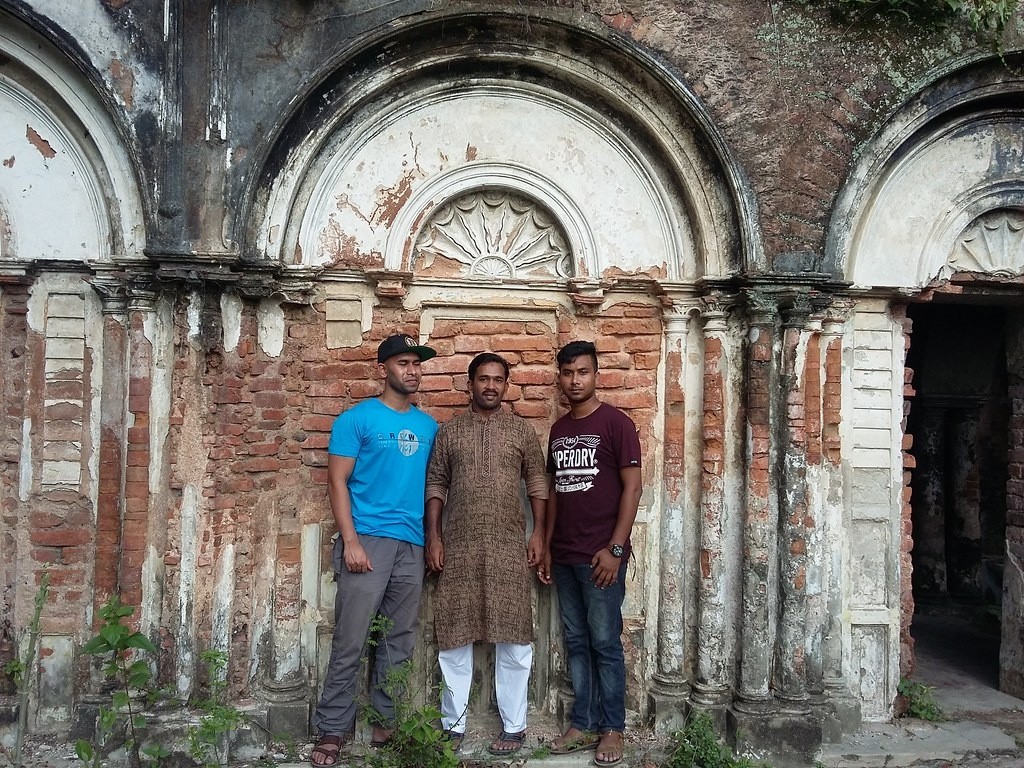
424, 353, 550, 755
535, 340, 643, 768
309, 333, 439, 768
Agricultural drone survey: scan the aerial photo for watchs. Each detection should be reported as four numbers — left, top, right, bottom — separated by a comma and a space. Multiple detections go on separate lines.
605, 543, 623, 558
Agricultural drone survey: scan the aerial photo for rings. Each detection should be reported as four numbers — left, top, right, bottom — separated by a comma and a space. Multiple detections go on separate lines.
536, 571, 542, 575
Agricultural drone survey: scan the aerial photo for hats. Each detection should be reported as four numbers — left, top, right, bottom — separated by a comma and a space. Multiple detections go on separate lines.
378, 334, 437, 363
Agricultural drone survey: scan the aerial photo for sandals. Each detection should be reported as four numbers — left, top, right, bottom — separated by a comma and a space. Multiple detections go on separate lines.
594, 730, 625, 766
548, 731, 602, 754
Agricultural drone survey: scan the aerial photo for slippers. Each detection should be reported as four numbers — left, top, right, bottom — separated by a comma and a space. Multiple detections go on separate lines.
491, 732, 525, 755
438, 731, 464, 753
369, 730, 419, 753
310, 732, 342, 768
